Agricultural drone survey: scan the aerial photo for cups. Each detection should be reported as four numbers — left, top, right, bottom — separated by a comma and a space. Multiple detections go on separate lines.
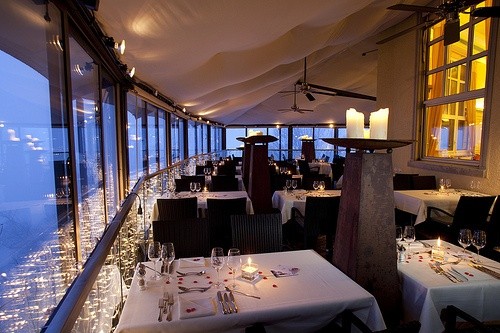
242, 263, 259, 282
431, 245, 447, 263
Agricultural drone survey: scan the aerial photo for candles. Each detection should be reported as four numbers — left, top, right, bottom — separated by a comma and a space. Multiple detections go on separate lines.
346, 107, 365, 138
368, 107, 389, 140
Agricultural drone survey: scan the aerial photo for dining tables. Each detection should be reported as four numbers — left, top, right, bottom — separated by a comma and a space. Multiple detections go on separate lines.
309, 162, 333, 181
235, 175, 246, 190
394, 187, 497, 226
271, 188, 342, 224
113, 248, 387, 333
395, 237, 500, 333
178, 190, 255, 218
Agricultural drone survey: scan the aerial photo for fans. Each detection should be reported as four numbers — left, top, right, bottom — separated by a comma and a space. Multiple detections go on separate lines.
277, 85, 314, 116
278, 56, 336, 101
374, 0, 500, 47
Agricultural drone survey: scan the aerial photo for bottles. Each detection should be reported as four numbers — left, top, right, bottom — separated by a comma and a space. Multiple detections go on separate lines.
136, 264, 146, 287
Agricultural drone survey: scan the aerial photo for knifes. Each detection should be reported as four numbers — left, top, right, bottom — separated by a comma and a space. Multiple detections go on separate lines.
473, 266, 500, 280
216, 290, 238, 314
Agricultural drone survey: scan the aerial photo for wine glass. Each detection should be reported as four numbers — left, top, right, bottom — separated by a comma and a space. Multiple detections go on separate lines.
210, 248, 225, 287
167, 161, 218, 199
286, 180, 297, 196
394, 226, 402, 252
313, 181, 325, 197
403, 226, 416, 256
468, 179, 483, 196
147, 242, 162, 280
439, 177, 452, 195
160, 242, 175, 283
471, 231, 486, 264
227, 249, 241, 289
458, 229, 472, 260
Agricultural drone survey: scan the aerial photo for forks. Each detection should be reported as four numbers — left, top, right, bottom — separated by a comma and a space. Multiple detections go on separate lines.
429, 263, 463, 283
157, 292, 174, 321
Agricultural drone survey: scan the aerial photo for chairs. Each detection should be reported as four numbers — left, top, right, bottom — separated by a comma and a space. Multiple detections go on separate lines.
152, 157, 500, 257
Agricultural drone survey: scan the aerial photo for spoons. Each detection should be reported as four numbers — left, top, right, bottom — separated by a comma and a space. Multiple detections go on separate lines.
175, 270, 206, 277
178, 285, 211, 293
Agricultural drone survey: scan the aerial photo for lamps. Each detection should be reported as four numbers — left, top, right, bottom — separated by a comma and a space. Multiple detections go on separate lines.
106, 37, 125, 54
121, 63, 136, 78
83, 0, 101, 12
